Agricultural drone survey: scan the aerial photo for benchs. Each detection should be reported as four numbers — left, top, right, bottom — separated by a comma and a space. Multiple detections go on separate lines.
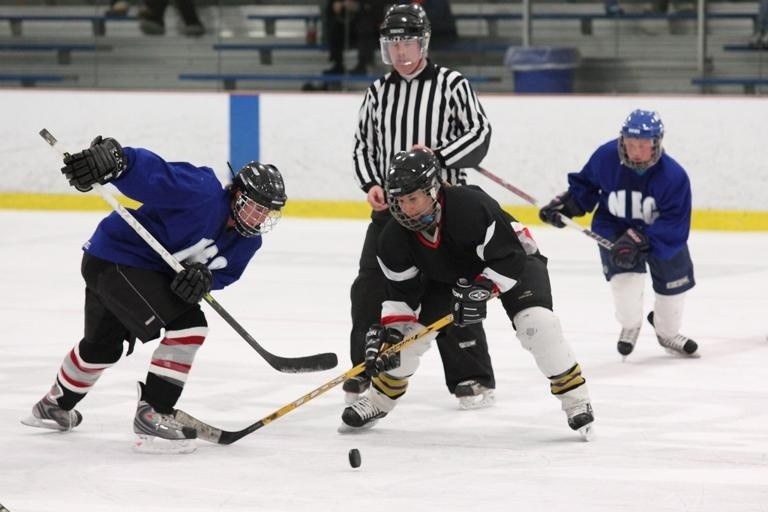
0, 1, 768, 96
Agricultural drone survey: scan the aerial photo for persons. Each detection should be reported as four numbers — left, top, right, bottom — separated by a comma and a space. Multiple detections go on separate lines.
604, 1, 669, 19
343, 3, 492, 407
104, 0, 205, 37
748, 2, 768, 49
341, 149, 593, 446
20, 136, 288, 455
301, 0, 458, 75
540, 110, 700, 357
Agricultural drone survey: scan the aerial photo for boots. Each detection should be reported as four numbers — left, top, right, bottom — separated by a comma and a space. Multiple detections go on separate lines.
338, 397, 389, 428
646, 307, 701, 356
616, 327, 641, 355
130, 378, 198, 442
560, 400, 596, 433
19, 380, 84, 429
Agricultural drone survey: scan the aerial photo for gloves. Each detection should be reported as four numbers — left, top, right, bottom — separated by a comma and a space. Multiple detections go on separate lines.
170, 262, 211, 307
363, 323, 401, 378
58, 134, 126, 186
537, 191, 585, 231
448, 277, 492, 328
607, 226, 651, 272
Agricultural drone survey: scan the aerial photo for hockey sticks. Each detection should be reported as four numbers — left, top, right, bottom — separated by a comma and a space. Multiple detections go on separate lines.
174, 313, 456, 446
39, 128, 338, 374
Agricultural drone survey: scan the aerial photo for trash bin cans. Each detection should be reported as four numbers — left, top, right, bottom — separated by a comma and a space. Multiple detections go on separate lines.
506, 45, 579, 94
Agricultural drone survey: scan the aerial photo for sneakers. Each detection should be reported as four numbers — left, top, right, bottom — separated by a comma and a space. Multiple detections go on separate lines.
342, 375, 372, 394
453, 379, 494, 400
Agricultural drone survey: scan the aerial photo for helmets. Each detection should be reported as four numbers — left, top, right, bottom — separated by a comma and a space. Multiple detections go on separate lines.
376, 2, 431, 72
616, 107, 664, 171
227, 160, 286, 241
383, 147, 443, 235
